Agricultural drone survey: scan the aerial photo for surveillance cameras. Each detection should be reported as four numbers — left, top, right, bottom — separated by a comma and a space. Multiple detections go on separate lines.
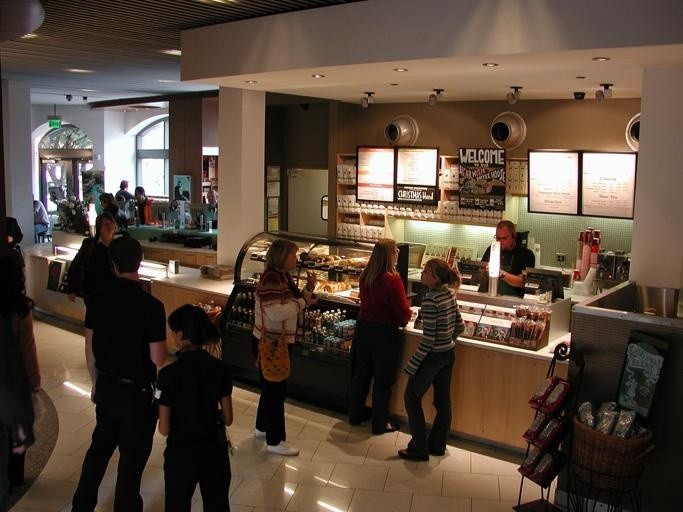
574, 91, 585, 101
65, 95, 73, 101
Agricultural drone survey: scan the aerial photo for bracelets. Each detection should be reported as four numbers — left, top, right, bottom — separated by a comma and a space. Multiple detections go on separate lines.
500, 270, 507, 280
305, 284, 314, 291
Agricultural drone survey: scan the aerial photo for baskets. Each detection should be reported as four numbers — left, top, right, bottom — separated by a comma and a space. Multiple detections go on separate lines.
571, 416, 653, 493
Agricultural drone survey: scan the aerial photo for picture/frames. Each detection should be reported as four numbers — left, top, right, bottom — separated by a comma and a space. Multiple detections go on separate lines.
172, 173, 192, 205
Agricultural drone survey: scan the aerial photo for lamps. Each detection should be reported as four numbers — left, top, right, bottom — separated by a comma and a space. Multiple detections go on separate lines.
427, 89, 444, 106
594, 83, 612, 104
488, 242, 500, 298
506, 86, 522, 104
360, 92, 375, 108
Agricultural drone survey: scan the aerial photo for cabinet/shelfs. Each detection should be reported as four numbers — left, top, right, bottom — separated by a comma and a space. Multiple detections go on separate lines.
213, 231, 404, 419
140, 239, 217, 271
336, 153, 516, 285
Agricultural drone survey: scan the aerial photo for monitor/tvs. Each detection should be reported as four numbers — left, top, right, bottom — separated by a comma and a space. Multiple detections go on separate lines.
456, 261, 489, 293
524, 267, 564, 300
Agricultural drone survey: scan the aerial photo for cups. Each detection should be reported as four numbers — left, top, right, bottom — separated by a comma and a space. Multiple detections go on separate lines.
487, 278, 499, 297
334, 164, 502, 242
570, 226, 602, 285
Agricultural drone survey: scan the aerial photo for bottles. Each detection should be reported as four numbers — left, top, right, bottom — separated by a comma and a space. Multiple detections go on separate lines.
228, 290, 256, 330
299, 306, 358, 361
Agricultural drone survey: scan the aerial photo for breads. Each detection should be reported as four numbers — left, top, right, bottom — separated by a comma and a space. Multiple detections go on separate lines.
252, 250, 267, 262
241, 272, 264, 283
299, 268, 360, 303
308, 254, 372, 268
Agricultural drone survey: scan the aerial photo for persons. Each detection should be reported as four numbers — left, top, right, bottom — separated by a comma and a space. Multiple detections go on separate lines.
0, 232, 40, 501
68, 210, 116, 403
30, 181, 156, 239
66, 236, 165, 511
155, 304, 232, 512
205, 189, 217, 251
351, 237, 412, 434
481, 219, 536, 299
250, 240, 316, 457
394, 258, 465, 460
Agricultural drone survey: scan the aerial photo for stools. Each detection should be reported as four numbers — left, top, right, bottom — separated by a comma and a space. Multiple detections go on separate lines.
38, 231, 52, 243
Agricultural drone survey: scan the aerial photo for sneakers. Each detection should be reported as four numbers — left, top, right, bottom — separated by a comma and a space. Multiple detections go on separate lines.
265, 440, 299, 456
398, 448, 412, 459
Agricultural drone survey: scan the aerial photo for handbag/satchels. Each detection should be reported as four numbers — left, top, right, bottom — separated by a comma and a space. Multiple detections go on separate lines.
259, 330, 292, 383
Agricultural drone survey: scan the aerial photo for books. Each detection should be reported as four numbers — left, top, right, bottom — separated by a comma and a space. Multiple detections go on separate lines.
199, 262, 241, 280
138, 277, 152, 296
45, 260, 68, 292
